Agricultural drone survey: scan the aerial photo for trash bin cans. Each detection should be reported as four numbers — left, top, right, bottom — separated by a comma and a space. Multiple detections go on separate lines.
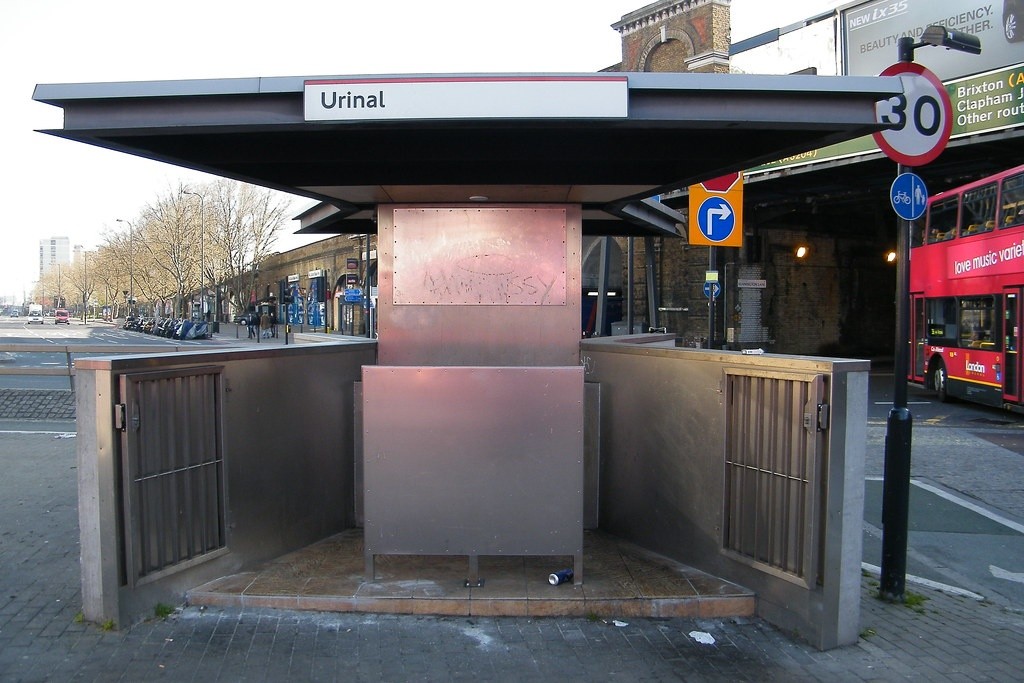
211, 322, 219, 332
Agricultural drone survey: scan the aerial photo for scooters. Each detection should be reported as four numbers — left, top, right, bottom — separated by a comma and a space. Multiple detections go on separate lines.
123, 317, 208, 340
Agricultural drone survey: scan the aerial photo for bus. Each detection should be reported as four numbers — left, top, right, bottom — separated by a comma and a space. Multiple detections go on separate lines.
907, 165, 1024, 415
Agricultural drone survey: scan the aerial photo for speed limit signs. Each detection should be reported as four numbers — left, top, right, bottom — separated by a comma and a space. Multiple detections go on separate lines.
871, 61, 953, 167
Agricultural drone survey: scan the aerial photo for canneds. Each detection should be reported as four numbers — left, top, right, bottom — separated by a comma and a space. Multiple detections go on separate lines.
548, 567, 574, 585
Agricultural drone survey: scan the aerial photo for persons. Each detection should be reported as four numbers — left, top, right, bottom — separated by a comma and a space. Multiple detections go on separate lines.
269, 312, 279, 337
246, 312, 255, 338
261, 312, 272, 340
255, 313, 261, 336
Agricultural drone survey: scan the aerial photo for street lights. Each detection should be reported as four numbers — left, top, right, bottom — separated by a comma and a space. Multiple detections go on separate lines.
184, 191, 204, 321
116, 219, 133, 318
878, 25, 981, 602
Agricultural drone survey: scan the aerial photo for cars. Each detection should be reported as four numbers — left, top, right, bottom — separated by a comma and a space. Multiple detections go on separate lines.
10, 310, 19, 318
233, 311, 276, 326
28, 310, 70, 325
